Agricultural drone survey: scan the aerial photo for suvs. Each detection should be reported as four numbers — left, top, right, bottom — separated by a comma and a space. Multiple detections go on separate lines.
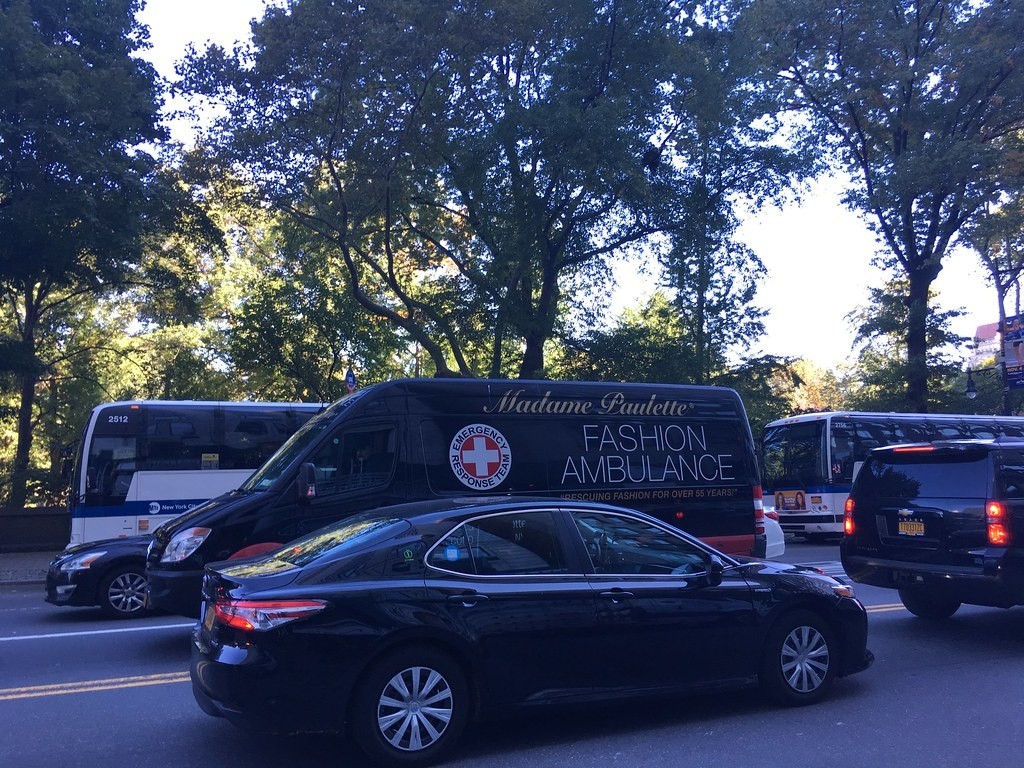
840, 436, 1023, 616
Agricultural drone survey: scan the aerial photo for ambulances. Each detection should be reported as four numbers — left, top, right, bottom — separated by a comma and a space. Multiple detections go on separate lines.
145, 374, 768, 560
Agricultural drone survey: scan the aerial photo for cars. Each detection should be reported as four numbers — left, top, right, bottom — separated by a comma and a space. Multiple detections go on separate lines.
187, 494, 875, 768
762, 506, 786, 560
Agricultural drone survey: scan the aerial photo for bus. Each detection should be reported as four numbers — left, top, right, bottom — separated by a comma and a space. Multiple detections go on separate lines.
64, 399, 333, 551
760, 408, 1024, 542
43, 534, 157, 619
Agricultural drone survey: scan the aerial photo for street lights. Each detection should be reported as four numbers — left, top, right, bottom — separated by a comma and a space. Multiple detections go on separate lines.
966, 365, 1013, 416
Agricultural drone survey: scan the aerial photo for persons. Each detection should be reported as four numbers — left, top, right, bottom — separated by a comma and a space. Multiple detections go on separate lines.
775, 491, 789, 510
790, 492, 806, 510
331, 434, 389, 490
